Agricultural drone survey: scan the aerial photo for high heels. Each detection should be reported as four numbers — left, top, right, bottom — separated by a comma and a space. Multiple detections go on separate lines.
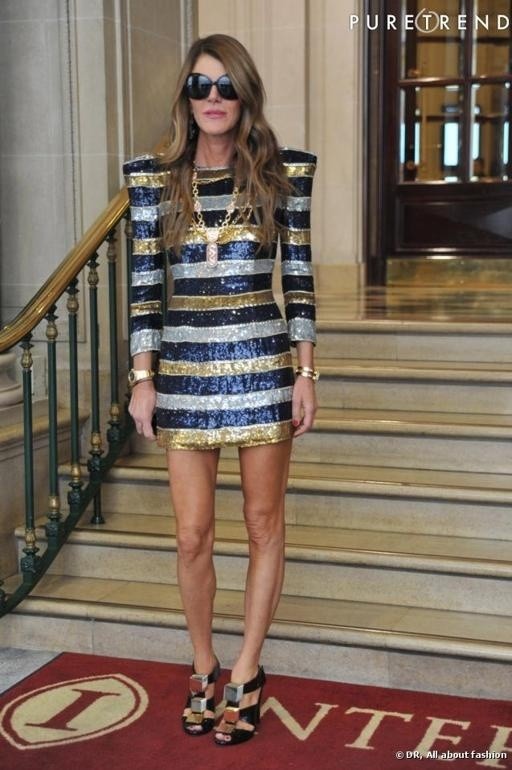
181, 654, 219, 737
213, 664, 267, 746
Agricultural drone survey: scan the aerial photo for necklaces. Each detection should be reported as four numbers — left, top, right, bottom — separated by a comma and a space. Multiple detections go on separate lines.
188, 161, 258, 273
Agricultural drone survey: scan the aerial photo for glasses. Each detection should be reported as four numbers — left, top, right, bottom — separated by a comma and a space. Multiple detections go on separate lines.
184, 73, 241, 100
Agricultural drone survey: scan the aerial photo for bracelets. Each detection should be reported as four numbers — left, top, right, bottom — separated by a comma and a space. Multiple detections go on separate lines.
295, 366, 320, 382
130, 379, 153, 388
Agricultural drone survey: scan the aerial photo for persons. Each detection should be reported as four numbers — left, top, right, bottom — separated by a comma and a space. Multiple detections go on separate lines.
405, 160, 427, 180
475, 158, 489, 178
123, 33, 312, 747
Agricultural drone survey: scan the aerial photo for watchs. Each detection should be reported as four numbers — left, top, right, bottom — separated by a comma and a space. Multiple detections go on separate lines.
123, 368, 156, 385
